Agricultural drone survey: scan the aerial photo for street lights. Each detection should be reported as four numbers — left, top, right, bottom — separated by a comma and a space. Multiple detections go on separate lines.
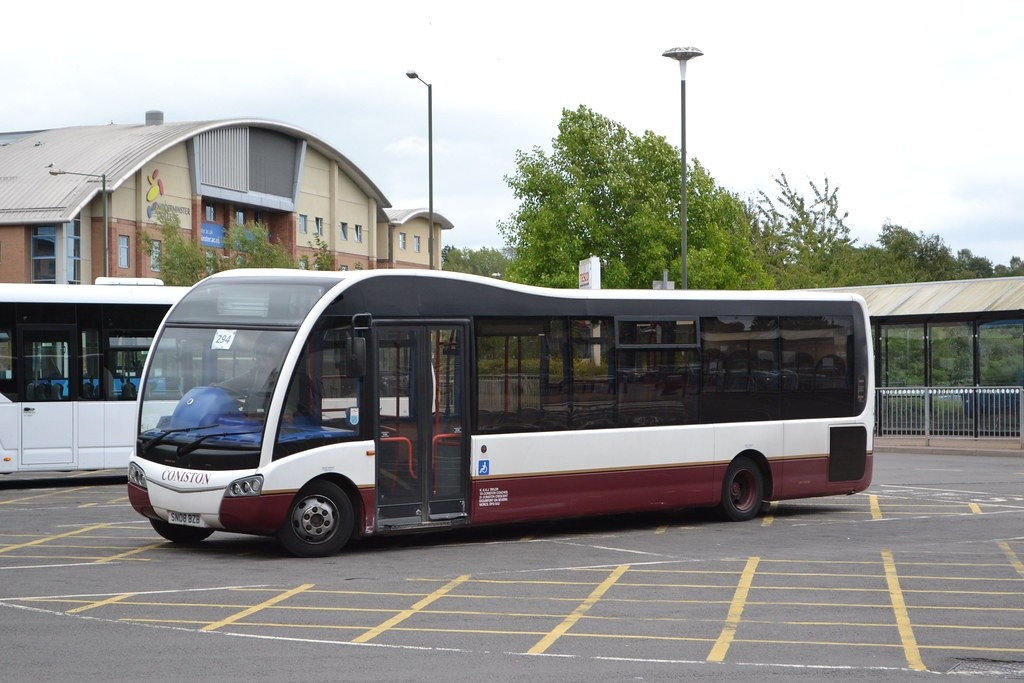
50, 169, 121, 281
406, 71, 439, 270
663, 47, 705, 290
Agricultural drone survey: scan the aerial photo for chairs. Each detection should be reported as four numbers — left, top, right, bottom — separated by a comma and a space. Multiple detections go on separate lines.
27, 382, 137, 401
296, 371, 315, 417
432, 348, 846, 434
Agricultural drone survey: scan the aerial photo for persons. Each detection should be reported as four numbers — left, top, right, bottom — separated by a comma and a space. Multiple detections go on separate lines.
209, 331, 299, 422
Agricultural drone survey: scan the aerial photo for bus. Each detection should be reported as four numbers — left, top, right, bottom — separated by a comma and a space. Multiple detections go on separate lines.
123, 267, 875, 557
0, 278, 445, 486
